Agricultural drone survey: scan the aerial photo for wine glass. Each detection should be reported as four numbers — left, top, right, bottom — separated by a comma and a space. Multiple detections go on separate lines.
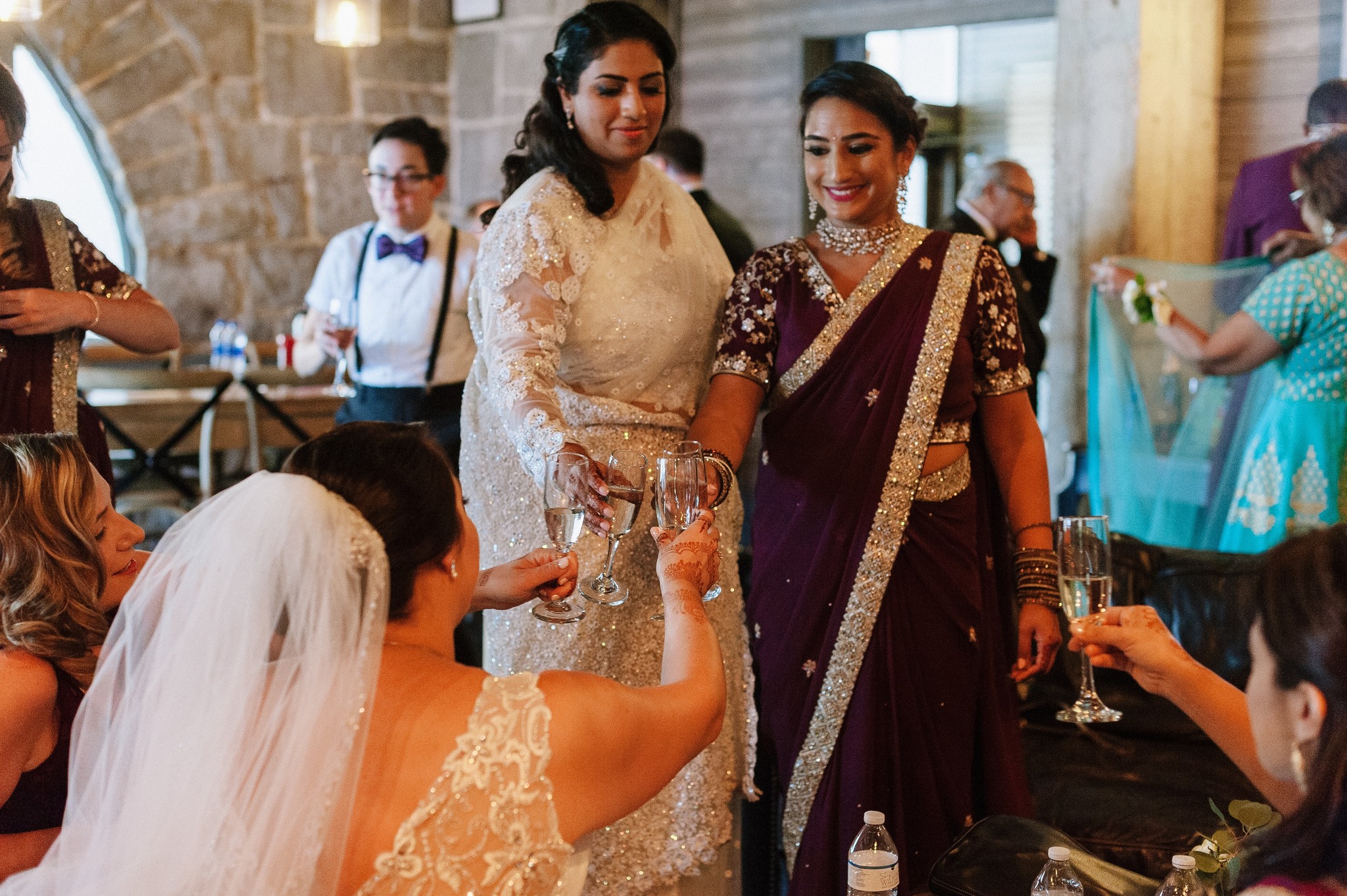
1054, 514, 1124, 723
577, 448, 649, 606
530, 452, 590, 624
651, 457, 698, 621
663, 440, 722, 602
323, 297, 358, 397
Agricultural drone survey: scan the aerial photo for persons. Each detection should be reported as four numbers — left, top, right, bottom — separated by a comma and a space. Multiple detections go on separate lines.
284, 112, 488, 463
1088, 138, 1346, 554
926, 160, 1059, 500
18, 416, 726, 896
660, 60, 1060, 892
1205, 76, 1345, 496
640, 124, 754, 273
445, 0, 740, 892
3, 433, 582, 878
0, 60, 180, 428
1062, 523, 1345, 895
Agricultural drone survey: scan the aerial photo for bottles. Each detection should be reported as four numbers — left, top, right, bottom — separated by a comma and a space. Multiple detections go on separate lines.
277, 335, 293, 370
209, 321, 247, 380
847, 811, 901, 896
1154, 854, 1206, 896
291, 309, 307, 341
1030, 847, 1085, 896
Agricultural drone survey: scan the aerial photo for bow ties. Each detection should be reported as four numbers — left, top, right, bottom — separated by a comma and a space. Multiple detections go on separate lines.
376, 232, 428, 262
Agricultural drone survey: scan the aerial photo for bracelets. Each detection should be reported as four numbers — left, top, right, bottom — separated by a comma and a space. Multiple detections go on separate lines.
1009, 546, 1068, 612
1006, 519, 1057, 545
690, 446, 737, 509
76, 290, 102, 331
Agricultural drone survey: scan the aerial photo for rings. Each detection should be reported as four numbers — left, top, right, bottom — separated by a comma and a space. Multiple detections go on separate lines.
715, 550, 724, 561
569, 493, 578, 503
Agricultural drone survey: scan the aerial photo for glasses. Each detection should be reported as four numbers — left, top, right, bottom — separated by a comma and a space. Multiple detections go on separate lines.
1288, 188, 1311, 207
996, 182, 1036, 207
363, 169, 435, 190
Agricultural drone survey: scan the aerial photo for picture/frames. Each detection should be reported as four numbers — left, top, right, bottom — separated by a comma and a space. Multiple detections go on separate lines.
447, 0, 504, 24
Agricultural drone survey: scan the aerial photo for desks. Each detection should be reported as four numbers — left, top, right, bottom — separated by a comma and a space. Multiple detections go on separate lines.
72, 381, 366, 506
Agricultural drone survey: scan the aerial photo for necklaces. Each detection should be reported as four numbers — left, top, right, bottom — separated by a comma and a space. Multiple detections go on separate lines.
380, 635, 453, 661
811, 214, 906, 256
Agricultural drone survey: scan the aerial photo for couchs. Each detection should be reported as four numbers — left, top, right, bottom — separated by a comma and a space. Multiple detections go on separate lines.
1014, 525, 1277, 877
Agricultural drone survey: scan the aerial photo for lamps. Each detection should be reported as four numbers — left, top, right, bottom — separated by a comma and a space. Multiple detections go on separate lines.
313, 1, 384, 48
